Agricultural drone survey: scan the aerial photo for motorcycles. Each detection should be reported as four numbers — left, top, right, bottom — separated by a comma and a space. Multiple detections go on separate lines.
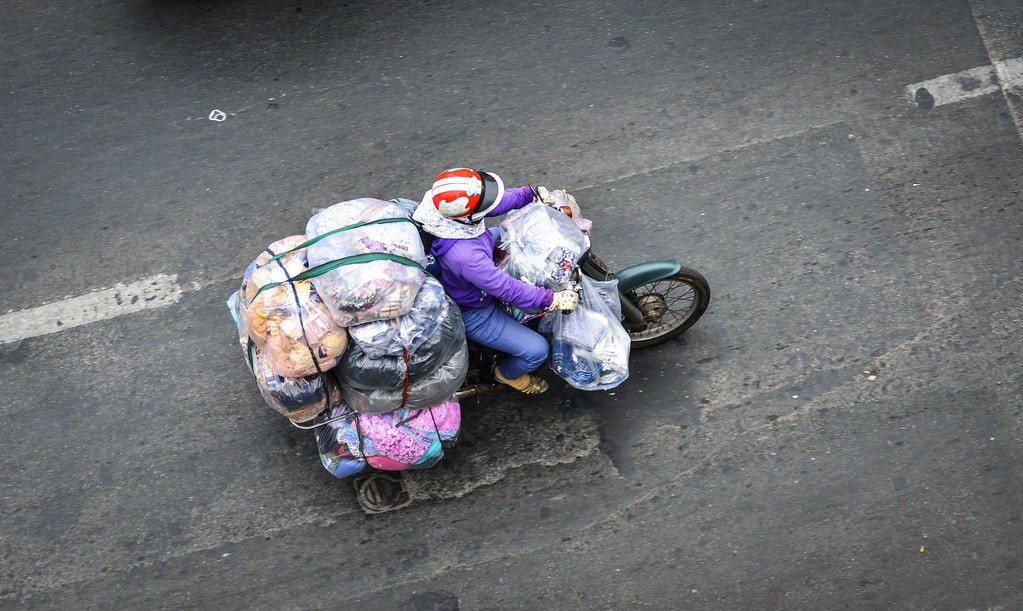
289, 184, 711, 429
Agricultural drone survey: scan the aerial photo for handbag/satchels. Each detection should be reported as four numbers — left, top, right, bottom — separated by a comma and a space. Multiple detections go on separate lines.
537, 265, 631, 392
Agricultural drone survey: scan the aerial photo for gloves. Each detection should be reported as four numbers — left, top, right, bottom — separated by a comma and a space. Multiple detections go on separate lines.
544, 290, 579, 312
533, 186, 556, 207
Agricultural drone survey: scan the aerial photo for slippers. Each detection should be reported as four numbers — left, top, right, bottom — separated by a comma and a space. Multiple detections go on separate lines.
495, 366, 548, 394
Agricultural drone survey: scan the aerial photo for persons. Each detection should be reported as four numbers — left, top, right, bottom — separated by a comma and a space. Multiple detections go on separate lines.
411, 168, 578, 395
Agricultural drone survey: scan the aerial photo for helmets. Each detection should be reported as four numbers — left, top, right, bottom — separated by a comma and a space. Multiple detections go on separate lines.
431, 167, 505, 224
